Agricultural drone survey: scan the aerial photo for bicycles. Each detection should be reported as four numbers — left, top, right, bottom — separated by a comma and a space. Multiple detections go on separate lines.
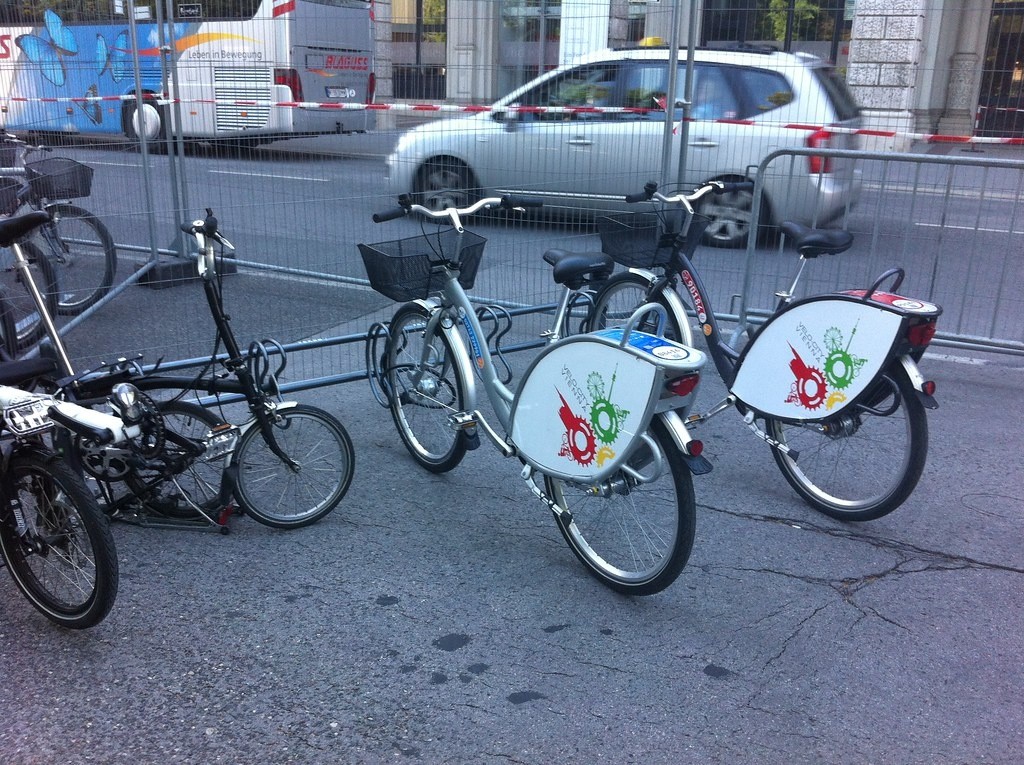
0, 130, 356, 632
598, 182, 948, 521
356, 195, 715, 598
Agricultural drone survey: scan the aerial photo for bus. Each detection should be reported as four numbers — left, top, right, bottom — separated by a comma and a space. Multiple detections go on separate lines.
1, 1, 375, 152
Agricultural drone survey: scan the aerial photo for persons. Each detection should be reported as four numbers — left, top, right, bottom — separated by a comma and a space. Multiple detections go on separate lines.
563, 86, 609, 121
685, 75, 726, 119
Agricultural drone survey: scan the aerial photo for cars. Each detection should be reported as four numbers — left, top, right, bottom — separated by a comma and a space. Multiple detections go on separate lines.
383, 44, 868, 248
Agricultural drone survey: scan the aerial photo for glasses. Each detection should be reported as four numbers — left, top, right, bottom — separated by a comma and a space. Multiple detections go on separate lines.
595, 87, 606, 92
